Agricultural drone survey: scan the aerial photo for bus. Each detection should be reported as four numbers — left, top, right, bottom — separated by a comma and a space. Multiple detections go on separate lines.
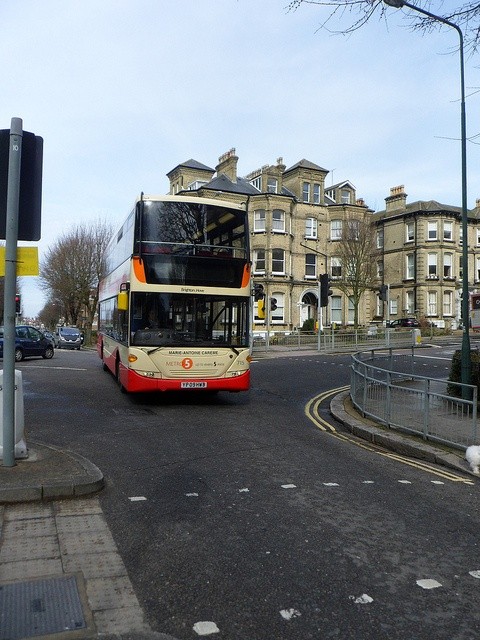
97, 193, 252, 395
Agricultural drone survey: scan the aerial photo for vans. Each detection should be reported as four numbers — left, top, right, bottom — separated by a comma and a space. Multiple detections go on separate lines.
387, 317, 421, 328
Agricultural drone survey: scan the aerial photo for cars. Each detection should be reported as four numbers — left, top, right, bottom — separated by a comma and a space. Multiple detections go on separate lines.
0, 325, 54, 361
44, 326, 84, 350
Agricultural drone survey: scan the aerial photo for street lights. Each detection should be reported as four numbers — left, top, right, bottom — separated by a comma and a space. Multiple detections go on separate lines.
383, 0, 479, 414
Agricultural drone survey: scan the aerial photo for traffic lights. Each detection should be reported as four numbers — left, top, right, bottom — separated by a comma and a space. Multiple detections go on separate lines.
320, 273, 332, 307
15, 294, 21, 314
254, 283, 263, 300
377, 282, 387, 302
270, 297, 279, 311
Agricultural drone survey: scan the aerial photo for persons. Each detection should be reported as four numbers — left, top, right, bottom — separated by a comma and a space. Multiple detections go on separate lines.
141, 308, 164, 329
